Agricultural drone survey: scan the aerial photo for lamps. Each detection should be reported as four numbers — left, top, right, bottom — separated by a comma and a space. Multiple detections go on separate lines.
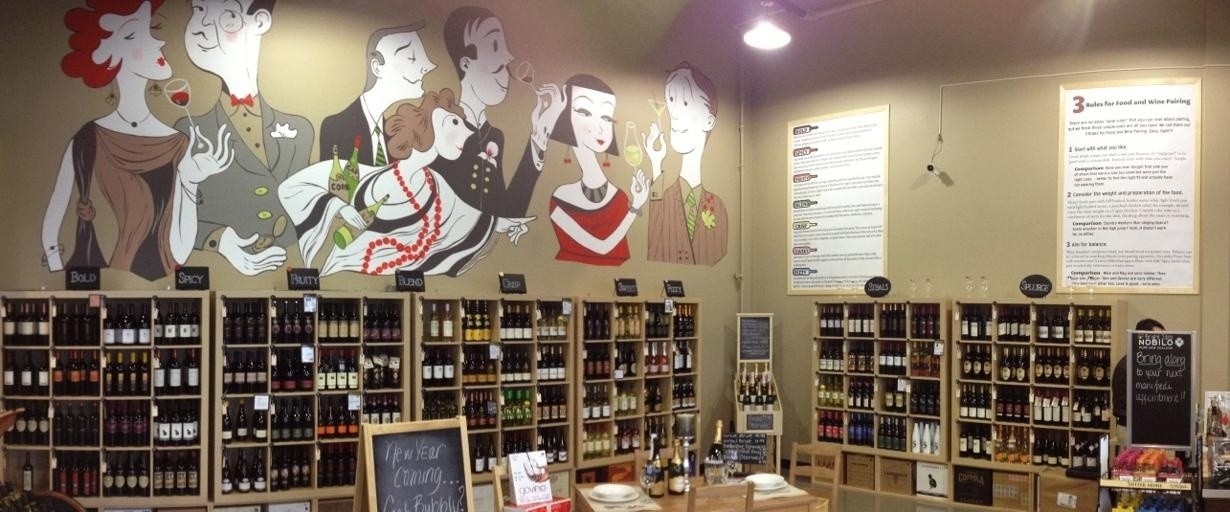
743, 0, 805, 49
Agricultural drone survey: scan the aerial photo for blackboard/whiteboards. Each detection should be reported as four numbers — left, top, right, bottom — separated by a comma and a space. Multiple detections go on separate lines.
736, 313, 773, 363
363, 416, 474, 512
1125, 329, 1197, 452
721, 432, 767, 465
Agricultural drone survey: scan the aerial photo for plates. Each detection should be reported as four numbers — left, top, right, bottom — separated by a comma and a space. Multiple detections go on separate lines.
589, 491, 639, 504
740, 481, 788, 492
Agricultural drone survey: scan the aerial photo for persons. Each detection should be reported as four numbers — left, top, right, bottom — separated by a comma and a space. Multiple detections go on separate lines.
1111, 318, 1166, 457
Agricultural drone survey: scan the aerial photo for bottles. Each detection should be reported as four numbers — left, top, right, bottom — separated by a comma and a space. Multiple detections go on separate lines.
583, 300, 698, 461
1208, 405, 1221, 436
645, 432, 656, 466
704, 419, 723, 484
959, 302, 1111, 473
648, 436, 665, 498
1111, 492, 1190, 512
421, 299, 569, 474
815, 302, 941, 456
152, 298, 202, 496
342, 134, 363, 197
327, 145, 350, 205
219, 297, 403, 496
791, 123, 822, 278
51, 297, 100, 497
1, 298, 51, 497
738, 370, 777, 410
333, 193, 389, 250
1111, 448, 1183, 482
102, 298, 151, 497
668, 439, 685, 495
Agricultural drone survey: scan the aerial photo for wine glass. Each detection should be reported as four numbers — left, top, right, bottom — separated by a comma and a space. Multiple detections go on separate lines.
638, 464, 657, 504
622, 119, 645, 192
705, 458, 725, 506
648, 99, 667, 136
515, 61, 551, 110
724, 447, 742, 489
163, 77, 207, 150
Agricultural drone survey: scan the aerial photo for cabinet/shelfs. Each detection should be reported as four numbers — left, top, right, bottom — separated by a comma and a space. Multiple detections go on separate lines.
730, 309, 783, 480
0, 293, 209, 512
413, 292, 576, 510
952, 297, 1120, 512
811, 296, 950, 512
575, 301, 698, 505
212, 291, 412, 509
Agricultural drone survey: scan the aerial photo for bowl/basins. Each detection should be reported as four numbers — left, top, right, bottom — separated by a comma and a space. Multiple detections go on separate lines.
594, 484, 634, 499
744, 472, 784, 487
